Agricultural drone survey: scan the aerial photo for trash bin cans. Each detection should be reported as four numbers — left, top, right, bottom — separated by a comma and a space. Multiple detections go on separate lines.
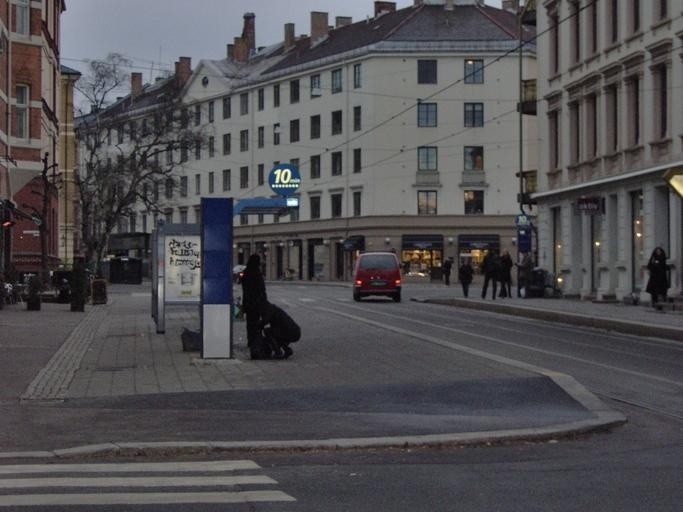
91, 280, 107, 306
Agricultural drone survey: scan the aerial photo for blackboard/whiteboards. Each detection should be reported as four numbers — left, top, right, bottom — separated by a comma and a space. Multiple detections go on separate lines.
430, 267, 442, 279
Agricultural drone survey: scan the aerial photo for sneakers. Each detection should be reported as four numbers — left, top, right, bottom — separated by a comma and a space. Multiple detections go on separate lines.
272, 346, 294, 360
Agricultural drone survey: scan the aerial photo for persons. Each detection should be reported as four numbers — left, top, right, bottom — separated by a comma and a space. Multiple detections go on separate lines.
261, 300, 303, 362
241, 253, 267, 347
646, 246, 668, 311
440, 244, 531, 300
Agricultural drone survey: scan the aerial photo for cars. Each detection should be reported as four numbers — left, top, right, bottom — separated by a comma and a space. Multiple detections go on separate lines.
352, 251, 402, 302
232, 263, 246, 285
